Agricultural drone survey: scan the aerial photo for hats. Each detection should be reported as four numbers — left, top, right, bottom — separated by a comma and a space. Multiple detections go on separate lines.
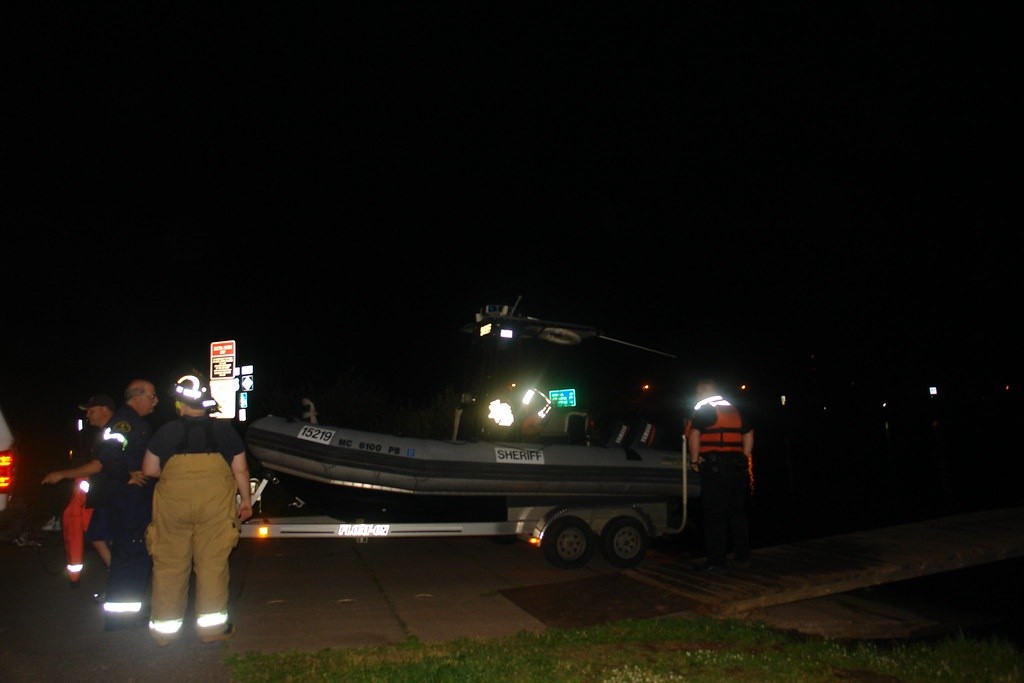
79, 395, 117, 411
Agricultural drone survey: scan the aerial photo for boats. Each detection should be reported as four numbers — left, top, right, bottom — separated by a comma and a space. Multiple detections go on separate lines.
246, 294, 706, 507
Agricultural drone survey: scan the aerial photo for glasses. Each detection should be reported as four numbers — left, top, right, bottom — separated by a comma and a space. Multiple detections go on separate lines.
143, 394, 156, 399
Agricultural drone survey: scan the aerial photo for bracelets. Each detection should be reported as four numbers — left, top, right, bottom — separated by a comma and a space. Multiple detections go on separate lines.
690, 461, 697, 465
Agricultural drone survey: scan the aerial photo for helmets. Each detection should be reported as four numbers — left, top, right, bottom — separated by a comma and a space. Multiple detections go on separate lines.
170, 373, 216, 409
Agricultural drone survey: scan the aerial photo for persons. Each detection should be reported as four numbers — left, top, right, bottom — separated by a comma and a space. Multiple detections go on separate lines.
61, 474, 112, 590
141, 362, 253, 646
41, 394, 116, 572
93, 381, 160, 630
685, 378, 756, 571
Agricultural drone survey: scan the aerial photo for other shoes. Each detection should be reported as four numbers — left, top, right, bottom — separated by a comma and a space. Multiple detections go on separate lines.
105, 615, 148, 631
148, 626, 169, 647
70, 579, 81, 589
200, 624, 234, 643
694, 561, 720, 576
736, 560, 752, 569
